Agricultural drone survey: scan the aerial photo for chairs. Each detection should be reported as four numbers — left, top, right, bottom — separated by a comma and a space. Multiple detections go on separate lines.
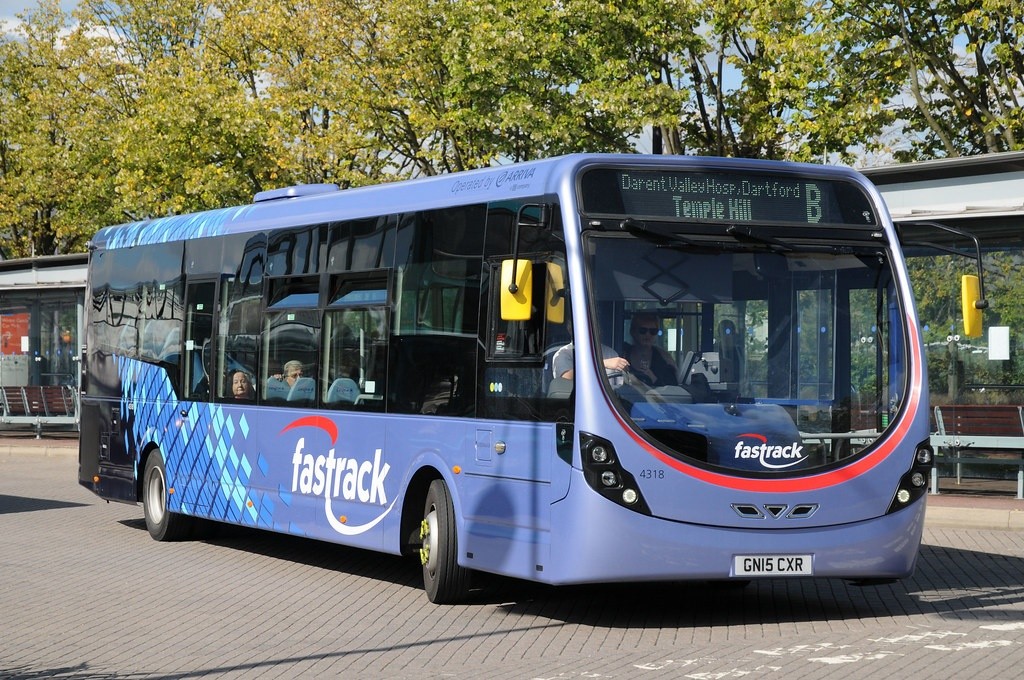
286, 377, 316, 402
326, 378, 364, 407
263, 376, 291, 400
121, 321, 256, 392
162, 351, 208, 399
541, 340, 572, 398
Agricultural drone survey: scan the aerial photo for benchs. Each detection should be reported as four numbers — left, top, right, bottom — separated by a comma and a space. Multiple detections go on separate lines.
829, 405, 1024, 499
0, 384, 80, 440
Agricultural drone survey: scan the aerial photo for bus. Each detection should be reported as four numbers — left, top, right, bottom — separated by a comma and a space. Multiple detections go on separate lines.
77, 153, 990, 606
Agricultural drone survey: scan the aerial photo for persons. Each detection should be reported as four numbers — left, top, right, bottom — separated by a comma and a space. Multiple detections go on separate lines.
232, 371, 250, 398
623, 315, 678, 389
547, 316, 631, 399
274, 360, 304, 382
713, 320, 746, 402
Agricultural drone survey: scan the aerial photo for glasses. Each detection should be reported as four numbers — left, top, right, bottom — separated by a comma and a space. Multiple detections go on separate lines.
285, 373, 303, 378
